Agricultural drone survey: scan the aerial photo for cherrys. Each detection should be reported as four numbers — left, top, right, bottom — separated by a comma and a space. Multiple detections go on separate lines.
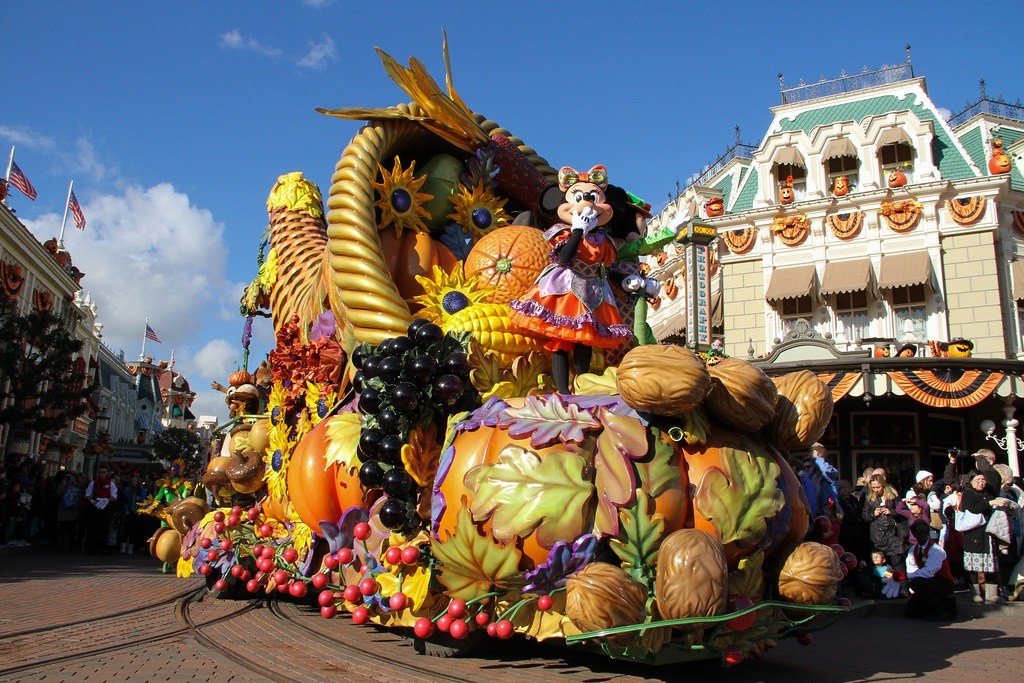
797, 515, 858, 646
201, 505, 566, 638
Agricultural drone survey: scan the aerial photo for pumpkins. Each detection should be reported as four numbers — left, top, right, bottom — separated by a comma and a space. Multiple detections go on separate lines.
228, 370, 251, 385
379, 227, 459, 313
285, 414, 754, 570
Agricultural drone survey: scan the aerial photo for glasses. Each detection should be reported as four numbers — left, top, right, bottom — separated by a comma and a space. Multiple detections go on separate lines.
99, 471, 107, 473
803, 465, 810, 471
1006, 481, 1014, 486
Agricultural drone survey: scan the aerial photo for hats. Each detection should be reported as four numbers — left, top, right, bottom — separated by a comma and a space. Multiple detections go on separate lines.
916, 470, 933, 483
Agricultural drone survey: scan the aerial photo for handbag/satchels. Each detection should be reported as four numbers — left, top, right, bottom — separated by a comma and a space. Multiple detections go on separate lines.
929, 511, 943, 530
955, 509, 986, 532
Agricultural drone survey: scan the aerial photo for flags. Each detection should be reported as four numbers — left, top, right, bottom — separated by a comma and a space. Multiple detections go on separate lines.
145, 325, 162, 343
68, 190, 86, 231
9, 160, 37, 201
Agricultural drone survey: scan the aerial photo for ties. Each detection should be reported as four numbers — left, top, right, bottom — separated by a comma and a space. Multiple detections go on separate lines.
917, 547, 923, 563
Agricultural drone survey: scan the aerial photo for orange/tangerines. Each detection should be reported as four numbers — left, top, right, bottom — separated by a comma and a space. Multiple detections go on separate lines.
462, 225, 555, 304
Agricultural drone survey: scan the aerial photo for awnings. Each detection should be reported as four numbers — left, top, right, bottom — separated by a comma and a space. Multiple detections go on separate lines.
764, 265, 816, 302
819, 258, 878, 301
153, 417, 166, 432
877, 251, 933, 295
134, 413, 151, 430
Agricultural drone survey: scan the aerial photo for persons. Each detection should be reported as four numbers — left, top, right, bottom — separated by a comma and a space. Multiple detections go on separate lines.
83, 464, 213, 556
787, 441, 1024, 623
508, 164, 646, 396
0, 453, 91, 552
603, 183, 690, 368
145, 458, 194, 574
220, 384, 259, 457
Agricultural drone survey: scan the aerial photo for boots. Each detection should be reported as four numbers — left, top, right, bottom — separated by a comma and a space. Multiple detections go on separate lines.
121, 542, 127, 553
127, 543, 133, 555
968, 583, 984, 603
985, 583, 1007, 605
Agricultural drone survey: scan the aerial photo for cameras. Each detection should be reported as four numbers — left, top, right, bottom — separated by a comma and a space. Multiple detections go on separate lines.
948, 447, 960, 459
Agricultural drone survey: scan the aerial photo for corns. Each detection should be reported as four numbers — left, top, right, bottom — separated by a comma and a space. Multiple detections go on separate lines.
487, 133, 553, 214
444, 303, 606, 375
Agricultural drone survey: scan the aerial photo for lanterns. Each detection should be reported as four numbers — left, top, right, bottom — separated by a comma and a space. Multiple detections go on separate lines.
949, 338, 974, 358
874, 346, 890, 358
892, 338, 916, 357
888, 170, 907, 188
704, 197, 723, 217
780, 184, 794, 204
989, 138, 1011, 175
833, 177, 849, 196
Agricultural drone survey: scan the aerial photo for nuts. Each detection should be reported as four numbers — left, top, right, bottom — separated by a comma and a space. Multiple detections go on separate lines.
564, 562, 649, 647
614, 345, 834, 453
654, 528, 731, 629
774, 541, 844, 606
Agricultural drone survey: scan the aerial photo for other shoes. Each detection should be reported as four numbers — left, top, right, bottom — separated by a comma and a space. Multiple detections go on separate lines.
953, 583, 970, 593
88, 549, 96, 555
99, 549, 114, 555
947, 606, 961, 619
923, 611, 944, 622
7, 539, 32, 546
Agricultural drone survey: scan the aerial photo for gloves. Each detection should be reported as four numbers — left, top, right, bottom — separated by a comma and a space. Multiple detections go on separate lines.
880, 576, 901, 598
95, 497, 109, 509
891, 570, 906, 581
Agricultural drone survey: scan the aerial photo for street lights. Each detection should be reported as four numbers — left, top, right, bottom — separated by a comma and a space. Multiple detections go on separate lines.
92, 406, 111, 481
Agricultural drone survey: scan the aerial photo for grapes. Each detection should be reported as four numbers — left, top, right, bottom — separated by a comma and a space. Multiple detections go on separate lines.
352, 319, 479, 534
